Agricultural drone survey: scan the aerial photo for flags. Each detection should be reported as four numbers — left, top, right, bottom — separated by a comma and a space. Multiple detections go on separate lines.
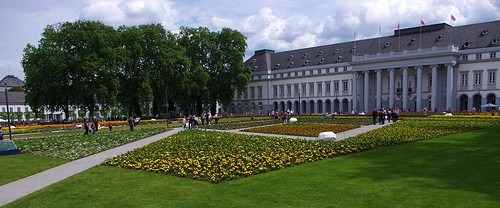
421, 19, 425, 26
396, 23, 400, 29
451, 15, 456, 21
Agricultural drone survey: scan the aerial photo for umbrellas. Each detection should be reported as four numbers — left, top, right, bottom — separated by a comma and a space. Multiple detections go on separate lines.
481, 103, 496, 107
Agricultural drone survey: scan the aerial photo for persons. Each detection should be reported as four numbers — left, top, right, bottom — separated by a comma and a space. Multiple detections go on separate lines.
182, 111, 219, 129
372, 105, 400, 125
94, 118, 99, 131
83, 117, 89, 136
270, 109, 293, 124
